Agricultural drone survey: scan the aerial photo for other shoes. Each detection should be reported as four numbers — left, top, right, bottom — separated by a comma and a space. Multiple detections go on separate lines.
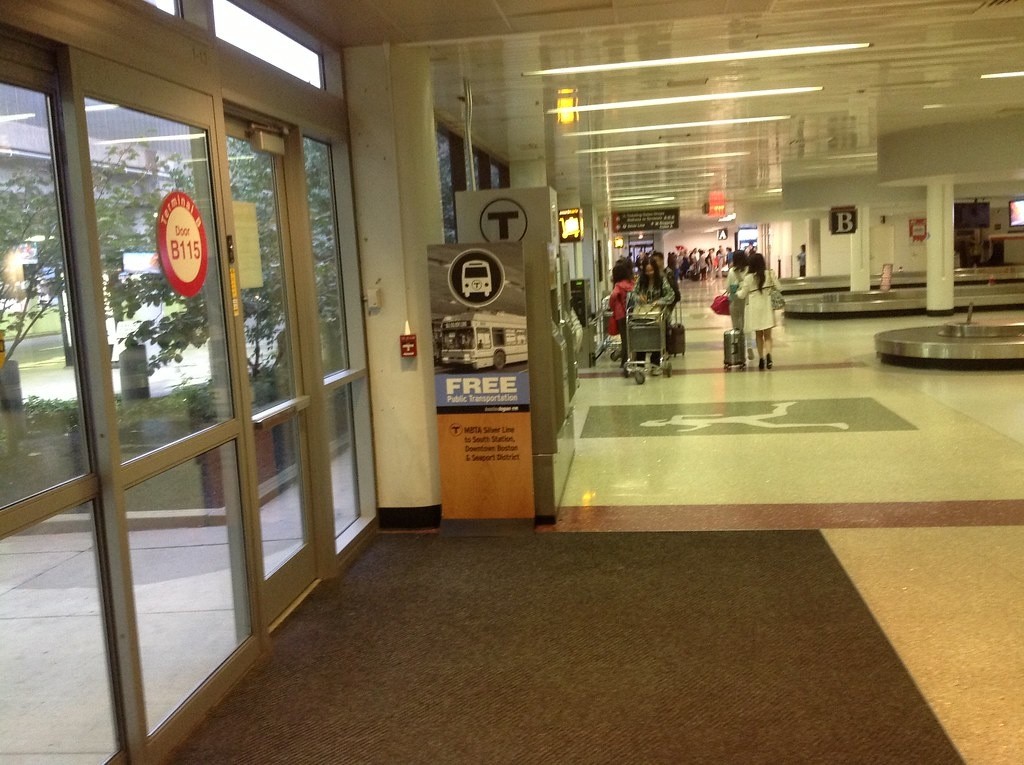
766, 353, 773, 369
759, 358, 765, 370
651, 365, 664, 375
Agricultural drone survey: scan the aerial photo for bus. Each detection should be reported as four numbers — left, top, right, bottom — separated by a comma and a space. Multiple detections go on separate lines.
439, 310, 527, 373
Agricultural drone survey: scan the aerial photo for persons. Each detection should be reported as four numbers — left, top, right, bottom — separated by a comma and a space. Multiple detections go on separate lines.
609, 241, 781, 375
796, 245, 806, 276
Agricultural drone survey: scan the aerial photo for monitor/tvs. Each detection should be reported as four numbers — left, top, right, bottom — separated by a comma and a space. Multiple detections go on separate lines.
1008, 199, 1024, 226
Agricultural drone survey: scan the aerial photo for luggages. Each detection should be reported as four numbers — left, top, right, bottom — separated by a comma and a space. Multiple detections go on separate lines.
724, 299, 747, 371
666, 301, 686, 357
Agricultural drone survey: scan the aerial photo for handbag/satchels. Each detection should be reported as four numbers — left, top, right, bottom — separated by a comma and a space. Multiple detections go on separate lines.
710, 290, 731, 315
769, 269, 785, 311
608, 311, 619, 335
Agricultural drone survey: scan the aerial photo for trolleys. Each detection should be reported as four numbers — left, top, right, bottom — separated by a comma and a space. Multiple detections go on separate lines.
623, 301, 674, 382
601, 294, 623, 360
